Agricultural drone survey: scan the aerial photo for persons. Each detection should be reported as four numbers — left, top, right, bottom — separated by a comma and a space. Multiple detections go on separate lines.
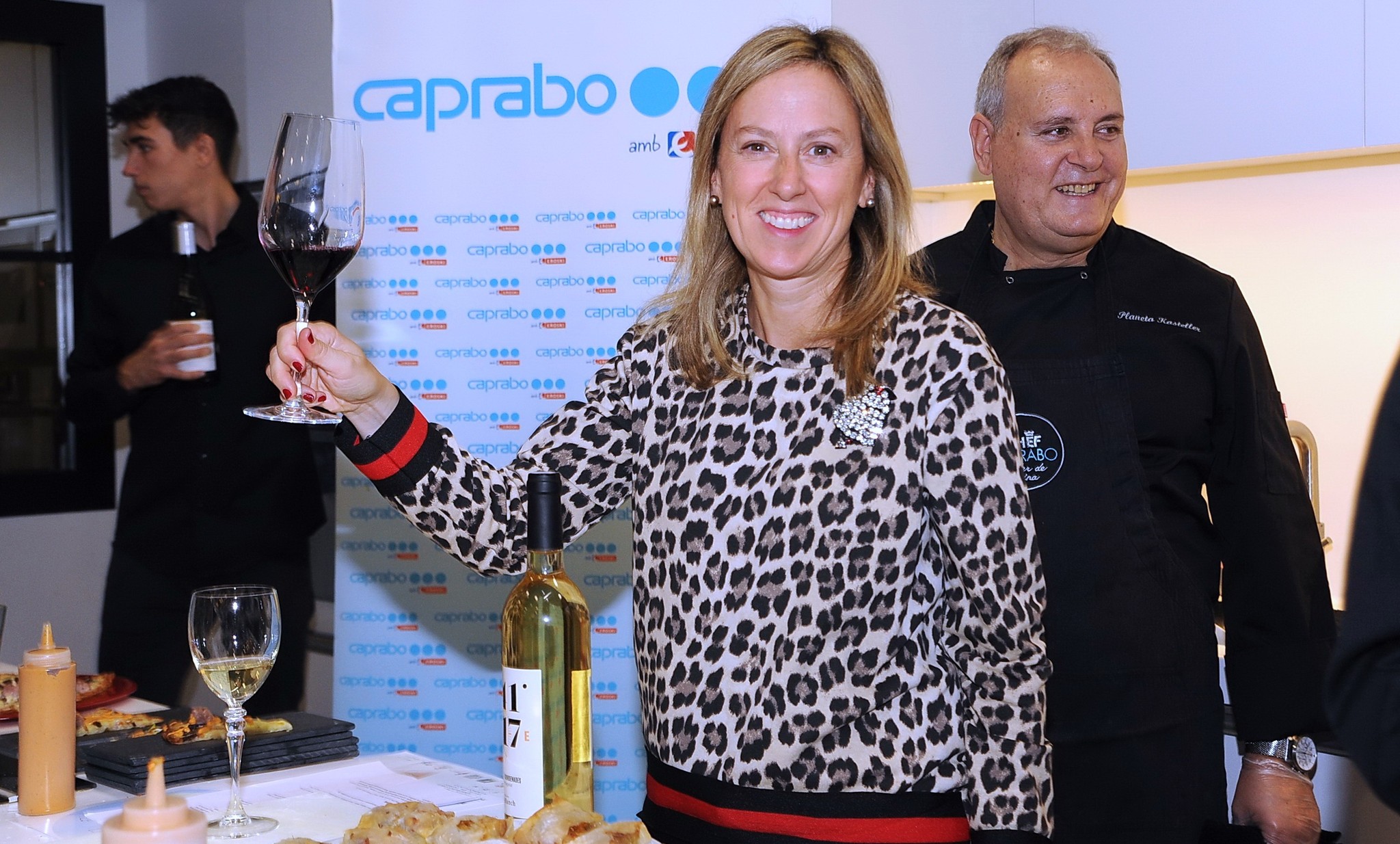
900, 26, 1334, 844
65, 74, 336, 719
1336, 348, 1400, 844
266, 24, 1054, 844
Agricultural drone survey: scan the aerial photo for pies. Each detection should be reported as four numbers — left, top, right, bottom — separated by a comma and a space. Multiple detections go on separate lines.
343, 801, 515, 844
508, 799, 654, 844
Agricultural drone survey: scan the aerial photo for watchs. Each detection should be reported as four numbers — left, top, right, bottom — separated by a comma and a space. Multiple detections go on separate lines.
1242, 736, 1318, 780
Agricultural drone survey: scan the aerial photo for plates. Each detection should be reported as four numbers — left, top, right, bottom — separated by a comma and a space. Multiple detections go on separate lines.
0, 673, 139, 719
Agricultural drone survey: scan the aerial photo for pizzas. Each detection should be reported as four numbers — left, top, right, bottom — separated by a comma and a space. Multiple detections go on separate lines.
76, 673, 115, 702
162, 707, 293, 744
76, 708, 164, 737
0, 673, 19, 713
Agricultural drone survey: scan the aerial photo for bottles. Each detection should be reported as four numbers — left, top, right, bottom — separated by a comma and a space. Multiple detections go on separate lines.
17, 621, 76, 817
100, 757, 208, 844
162, 219, 217, 392
500, 470, 594, 825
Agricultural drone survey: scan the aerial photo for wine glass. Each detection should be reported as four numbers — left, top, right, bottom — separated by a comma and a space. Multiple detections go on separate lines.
186, 584, 281, 841
242, 112, 366, 425
